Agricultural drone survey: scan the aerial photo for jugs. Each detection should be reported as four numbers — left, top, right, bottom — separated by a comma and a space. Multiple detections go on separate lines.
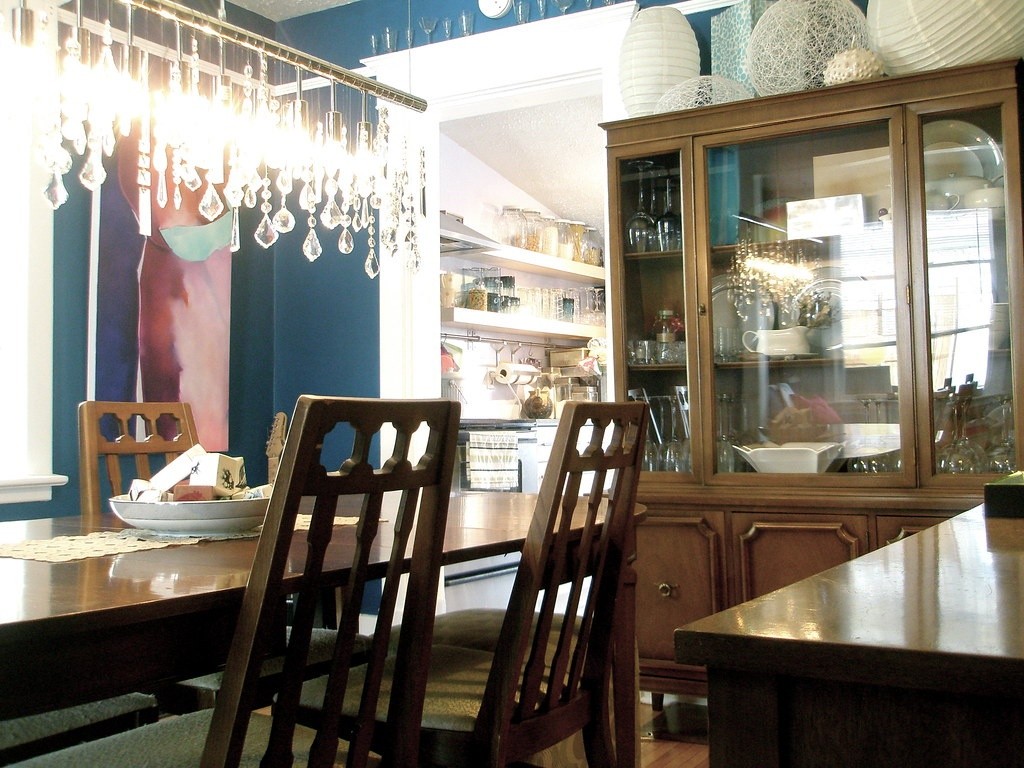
742, 326, 813, 354
926, 194, 961, 211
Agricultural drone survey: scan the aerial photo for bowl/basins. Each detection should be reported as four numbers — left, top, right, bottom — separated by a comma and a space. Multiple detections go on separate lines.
109, 487, 269, 538
732, 441, 849, 476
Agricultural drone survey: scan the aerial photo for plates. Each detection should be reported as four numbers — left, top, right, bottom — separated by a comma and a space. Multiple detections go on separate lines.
922, 118, 1005, 193
745, 352, 820, 360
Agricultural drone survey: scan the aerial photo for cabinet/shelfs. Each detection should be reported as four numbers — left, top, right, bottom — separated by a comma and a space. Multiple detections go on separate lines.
597, 57, 1024, 711
441, 243, 605, 341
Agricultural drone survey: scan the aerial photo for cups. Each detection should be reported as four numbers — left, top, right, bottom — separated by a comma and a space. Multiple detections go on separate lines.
625, 340, 685, 365
713, 326, 740, 363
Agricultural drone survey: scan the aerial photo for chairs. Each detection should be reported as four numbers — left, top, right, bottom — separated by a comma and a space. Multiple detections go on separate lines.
0, 391, 650, 768
931, 372, 978, 452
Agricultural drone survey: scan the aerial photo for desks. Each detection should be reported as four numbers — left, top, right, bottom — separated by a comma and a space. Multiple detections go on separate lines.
0, 490, 648, 768
674, 503, 1024, 768
834, 422, 900, 459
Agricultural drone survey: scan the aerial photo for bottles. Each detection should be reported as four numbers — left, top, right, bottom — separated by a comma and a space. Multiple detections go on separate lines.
652, 310, 676, 343
497, 206, 601, 266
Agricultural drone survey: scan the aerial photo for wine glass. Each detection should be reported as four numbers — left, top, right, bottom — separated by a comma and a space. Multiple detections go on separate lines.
581, 287, 596, 326
937, 394, 1016, 474
593, 288, 605, 328
629, 395, 740, 472
623, 158, 683, 253
